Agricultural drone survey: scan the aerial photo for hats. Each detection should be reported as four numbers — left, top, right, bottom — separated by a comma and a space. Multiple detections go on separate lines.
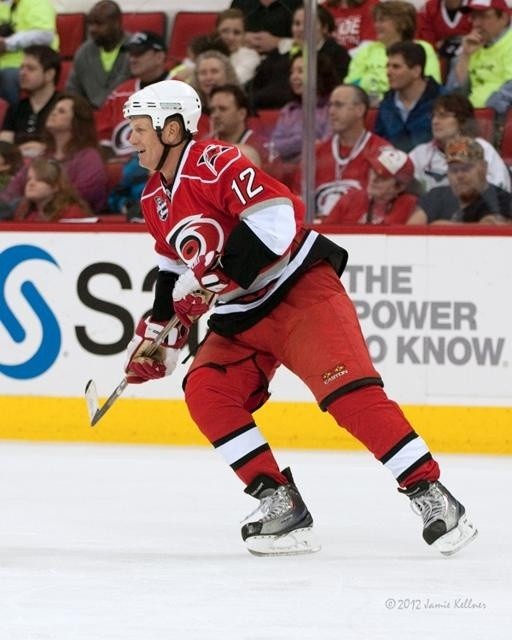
121, 31, 163, 51
460, 0, 507, 13
445, 137, 483, 163
366, 146, 413, 180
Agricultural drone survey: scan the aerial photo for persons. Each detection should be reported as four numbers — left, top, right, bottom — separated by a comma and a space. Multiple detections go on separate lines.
118, 76, 468, 546
2, 0, 512, 228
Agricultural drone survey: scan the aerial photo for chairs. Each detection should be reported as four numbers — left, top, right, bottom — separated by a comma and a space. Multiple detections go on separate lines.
0, 0, 512, 225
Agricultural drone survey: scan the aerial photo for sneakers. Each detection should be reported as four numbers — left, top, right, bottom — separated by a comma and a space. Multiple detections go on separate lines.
398, 480, 465, 544
242, 466, 312, 540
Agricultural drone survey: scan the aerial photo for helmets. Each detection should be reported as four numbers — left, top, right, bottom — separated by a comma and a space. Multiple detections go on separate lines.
122, 80, 202, 134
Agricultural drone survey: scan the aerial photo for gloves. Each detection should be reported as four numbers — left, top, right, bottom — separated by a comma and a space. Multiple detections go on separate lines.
172, 251, 229, 328
123, 315, 190, 383
